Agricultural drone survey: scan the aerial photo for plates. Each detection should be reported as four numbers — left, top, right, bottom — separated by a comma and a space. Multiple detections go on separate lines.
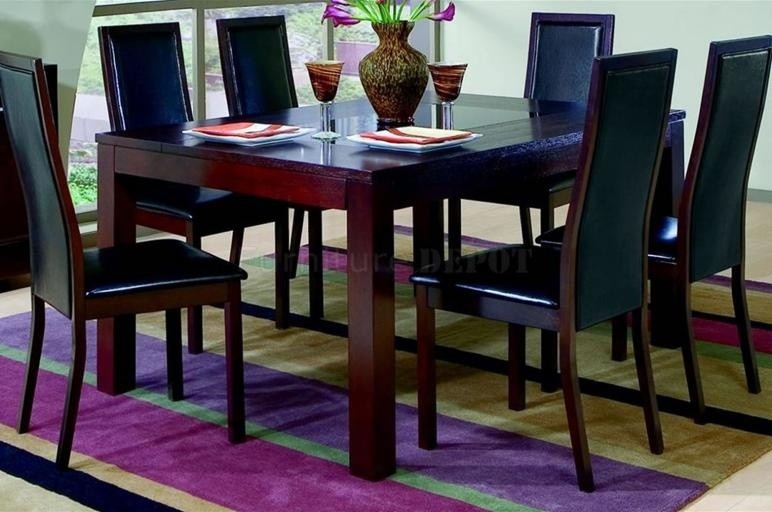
346, 125, 485, 156
183, 127, 315, 147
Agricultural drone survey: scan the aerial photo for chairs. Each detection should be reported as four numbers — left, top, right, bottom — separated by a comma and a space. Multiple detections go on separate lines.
214, 13, 398, 319
533, 36, 771, 428
445, 12, 615, 261
408, 43, 680, 494
1, 48, 247, 476
96, 20, 291, 352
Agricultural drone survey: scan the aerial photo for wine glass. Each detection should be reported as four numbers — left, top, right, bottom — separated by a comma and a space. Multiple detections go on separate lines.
307, 59, 344, 139
427, 61, 468, 129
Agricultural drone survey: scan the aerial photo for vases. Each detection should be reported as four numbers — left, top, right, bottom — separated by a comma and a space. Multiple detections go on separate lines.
357, 20, 431, 127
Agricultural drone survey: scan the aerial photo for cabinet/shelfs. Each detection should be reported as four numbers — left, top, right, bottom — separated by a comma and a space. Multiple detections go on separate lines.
0, 61, 59, 294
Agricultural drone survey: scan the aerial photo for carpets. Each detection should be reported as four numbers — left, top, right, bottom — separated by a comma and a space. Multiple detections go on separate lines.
0, 223, 771, 512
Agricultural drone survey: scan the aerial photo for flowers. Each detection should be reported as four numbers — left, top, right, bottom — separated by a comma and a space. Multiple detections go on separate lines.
322, 2, 457, 30
